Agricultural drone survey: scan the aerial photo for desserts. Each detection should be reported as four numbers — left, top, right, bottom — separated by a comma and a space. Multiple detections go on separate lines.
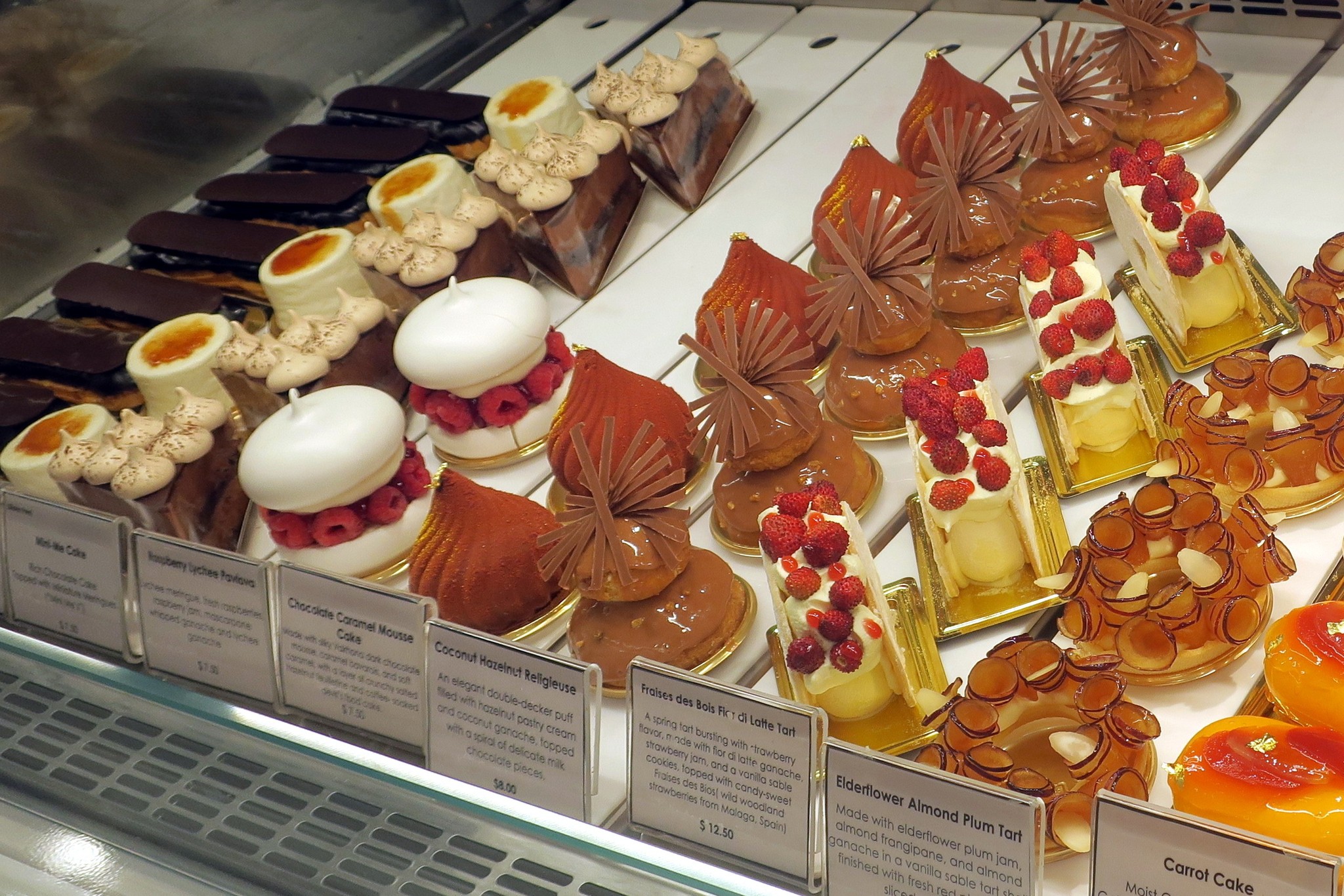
0, 0, 1344, 885
